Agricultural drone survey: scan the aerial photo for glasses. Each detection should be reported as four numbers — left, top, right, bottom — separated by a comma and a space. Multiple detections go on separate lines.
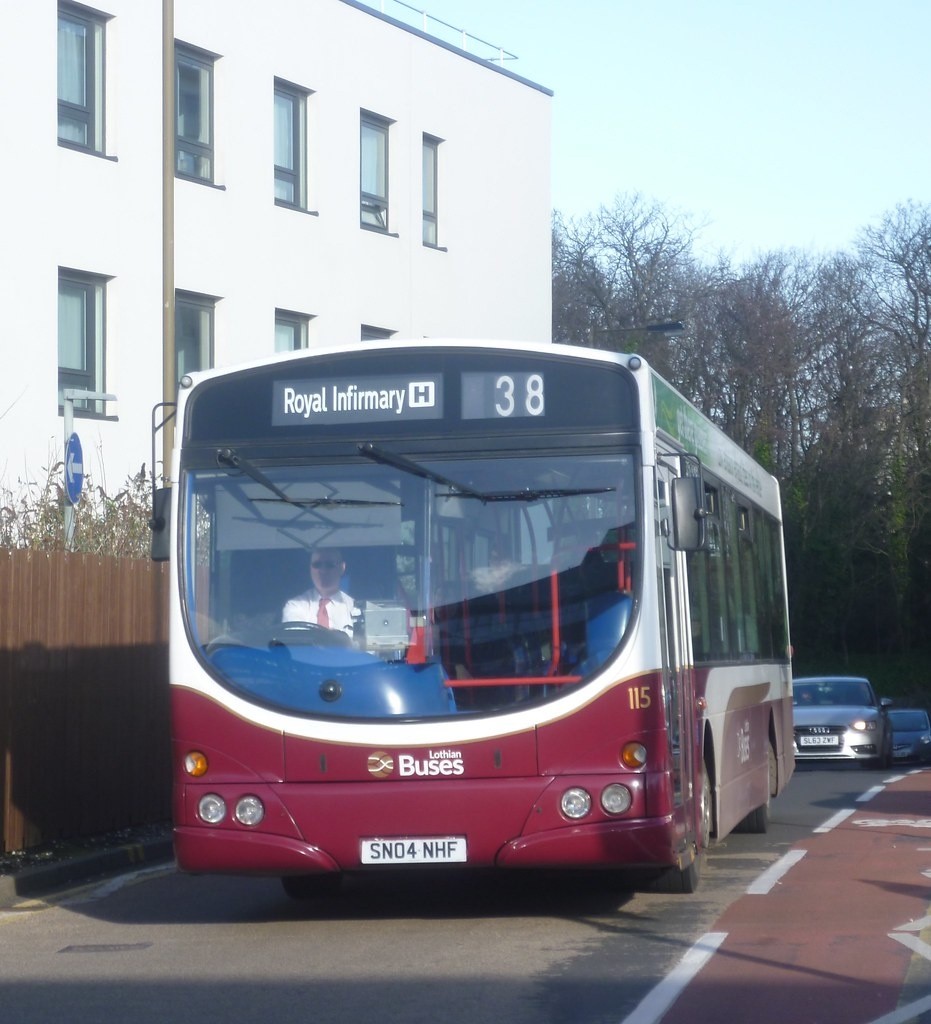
310, 560, 342, 568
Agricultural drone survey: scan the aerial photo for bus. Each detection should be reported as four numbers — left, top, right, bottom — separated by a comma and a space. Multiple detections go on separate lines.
146, 340, 795, 897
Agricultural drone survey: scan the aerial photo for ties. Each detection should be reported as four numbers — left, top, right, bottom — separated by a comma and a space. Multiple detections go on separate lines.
317, 597, 330, 629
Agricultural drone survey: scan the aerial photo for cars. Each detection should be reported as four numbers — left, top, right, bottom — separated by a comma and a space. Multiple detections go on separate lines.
886, 708, 931, 768
793, 676, 893, 770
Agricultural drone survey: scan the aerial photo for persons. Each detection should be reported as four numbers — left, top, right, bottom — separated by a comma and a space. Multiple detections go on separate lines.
280, 545, 362, 643
798, 690, 814, 705
433, 580, 461, 624
